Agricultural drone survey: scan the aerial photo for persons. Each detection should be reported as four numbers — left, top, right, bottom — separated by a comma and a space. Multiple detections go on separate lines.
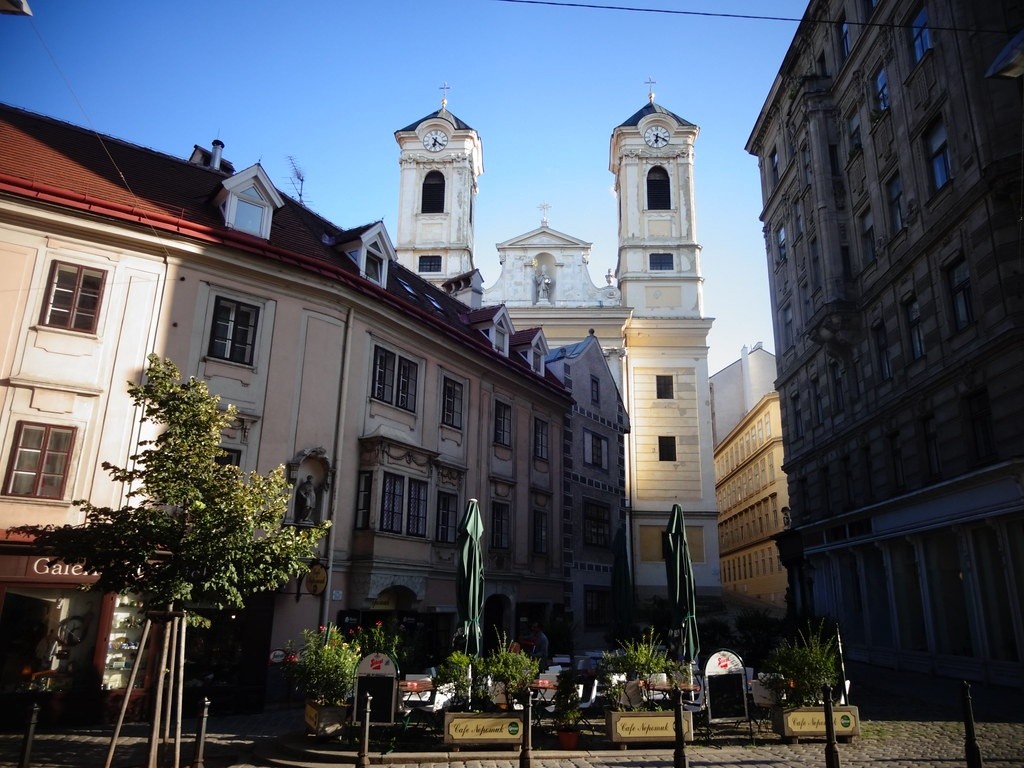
536, 265, 551, 305
537, 630, 548, 657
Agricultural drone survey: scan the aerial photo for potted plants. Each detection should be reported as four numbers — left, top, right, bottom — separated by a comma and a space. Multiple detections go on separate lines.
770, 617, 862, 745
436, 623, 542, 749
594, 624, 696, 750
552, 668, 586, 749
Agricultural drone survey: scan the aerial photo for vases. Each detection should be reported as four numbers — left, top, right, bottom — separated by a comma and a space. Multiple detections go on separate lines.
303, 701, 349, 743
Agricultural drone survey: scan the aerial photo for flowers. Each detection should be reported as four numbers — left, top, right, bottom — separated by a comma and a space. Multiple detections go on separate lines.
282, 622, 363, 706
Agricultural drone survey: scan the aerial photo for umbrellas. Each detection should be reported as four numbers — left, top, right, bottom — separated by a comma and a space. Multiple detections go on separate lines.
452, 498, 484, 712
666, 503, 701, 701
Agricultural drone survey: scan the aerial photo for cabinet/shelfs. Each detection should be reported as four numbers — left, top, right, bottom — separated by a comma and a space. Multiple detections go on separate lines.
92, 590, 160, 725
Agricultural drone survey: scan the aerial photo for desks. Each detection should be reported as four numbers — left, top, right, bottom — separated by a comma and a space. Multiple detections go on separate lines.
645, 683, 701, 710
401, 679, 437, 733
512, 685, 558, 735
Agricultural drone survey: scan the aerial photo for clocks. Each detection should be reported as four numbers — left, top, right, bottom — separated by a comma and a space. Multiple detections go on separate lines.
422, 129, 448, 152
644, 125, 670, 149
